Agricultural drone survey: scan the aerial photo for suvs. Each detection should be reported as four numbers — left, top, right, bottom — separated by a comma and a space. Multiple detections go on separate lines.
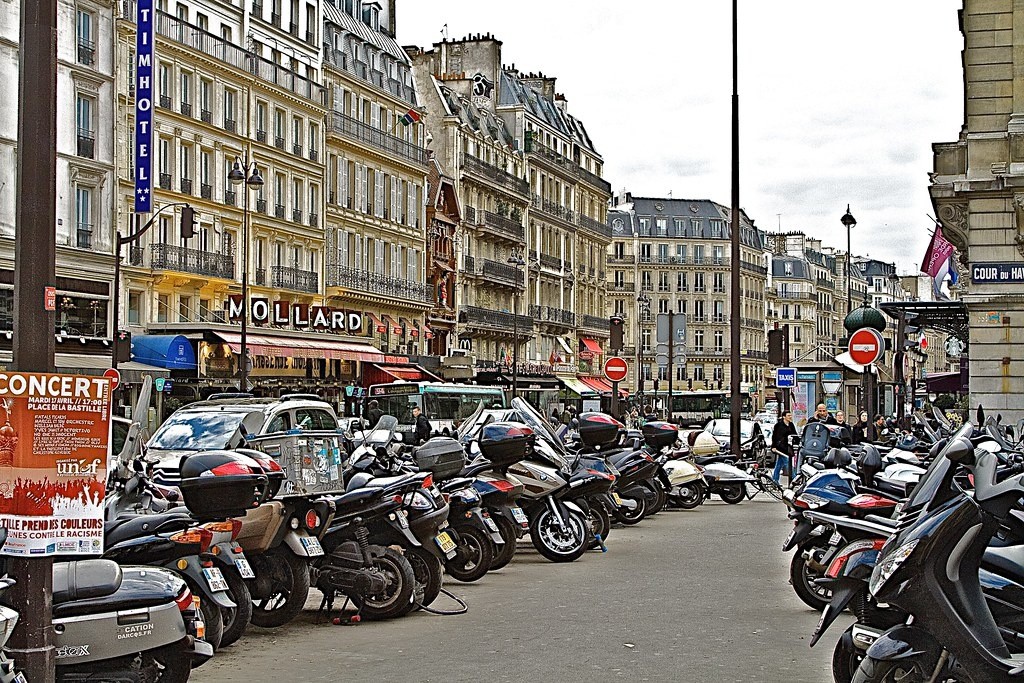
140, 391, 340, 494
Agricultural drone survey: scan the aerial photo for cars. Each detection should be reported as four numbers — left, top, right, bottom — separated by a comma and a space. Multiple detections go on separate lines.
702, 418, 767, 467
111, 416, 135, 462
754, 402, 784, 464
459, 408, 542, 436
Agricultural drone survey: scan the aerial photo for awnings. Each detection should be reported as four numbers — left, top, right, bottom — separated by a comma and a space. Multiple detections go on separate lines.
366, 312, 386, 333
212, 330, 385, 363
414, 319, 433, 339
556, 374, 629, 399
555, 337, 573, 354
381, 314, 402, 334
399, 317, 418, 336
581, 337, 604, 354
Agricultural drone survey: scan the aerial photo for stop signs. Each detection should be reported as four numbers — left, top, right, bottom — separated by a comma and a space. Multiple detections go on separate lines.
103, 368, 121, 392
603, 358, 627, 382
753, 393, 758, 398
849, 330, 880, 366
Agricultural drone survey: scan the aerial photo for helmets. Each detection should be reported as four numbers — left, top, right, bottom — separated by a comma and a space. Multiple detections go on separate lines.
910, 440, 928, 452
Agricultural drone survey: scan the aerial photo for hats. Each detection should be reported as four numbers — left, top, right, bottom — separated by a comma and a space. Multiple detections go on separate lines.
905, 414, 912, 418
368, 400, 378, 405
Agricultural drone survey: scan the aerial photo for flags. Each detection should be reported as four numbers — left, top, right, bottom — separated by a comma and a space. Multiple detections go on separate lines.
549, 350, 563, 367
500, 347, 505, 360
919, 328, 927, 348
507, 349, 512, 364
400, 109, 422, 126
919, 224, 959, 300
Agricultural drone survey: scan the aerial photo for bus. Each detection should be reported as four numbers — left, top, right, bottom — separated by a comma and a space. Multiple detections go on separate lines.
366, 380, 508, 435
669, 390, 754, 428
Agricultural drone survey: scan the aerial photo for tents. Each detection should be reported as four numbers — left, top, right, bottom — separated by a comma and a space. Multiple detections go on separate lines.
926, 372, 961, 414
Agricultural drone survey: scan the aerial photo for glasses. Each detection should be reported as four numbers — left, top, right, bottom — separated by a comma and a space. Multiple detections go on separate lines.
819, 410, 826, 412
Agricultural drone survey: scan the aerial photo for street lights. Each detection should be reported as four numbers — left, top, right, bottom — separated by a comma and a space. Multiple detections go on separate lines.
636, 291, 649, 415
911, 347, 918, 411
507, 249, 526, 398
228, 154, 264, 391
888, 263, 900, 353
841, 205, 857, 340
932, 333, 937, 373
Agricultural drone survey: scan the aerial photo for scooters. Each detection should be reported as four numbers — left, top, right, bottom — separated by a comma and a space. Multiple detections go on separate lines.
0, 399, 761, 683
781, 405, 1024, 683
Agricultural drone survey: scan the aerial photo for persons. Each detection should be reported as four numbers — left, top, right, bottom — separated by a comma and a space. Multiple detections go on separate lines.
806, 403, 835, 446
452, 421, 462, 441
836, 410, 852, 439
552, 404, 576, 425
852, 410, 912, 446
771, 410, 800, 489
368, 400, 381, 430
620, 405, 658, 430
412, 406, 432, 446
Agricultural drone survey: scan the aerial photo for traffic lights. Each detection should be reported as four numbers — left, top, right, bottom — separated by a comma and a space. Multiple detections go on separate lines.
894, 383, 906, 395
703, 379, 708, 388
181, 207, 199, 240
687, 378, 693, 389
898, 310, 919, 354
709, 383, 713, 389
